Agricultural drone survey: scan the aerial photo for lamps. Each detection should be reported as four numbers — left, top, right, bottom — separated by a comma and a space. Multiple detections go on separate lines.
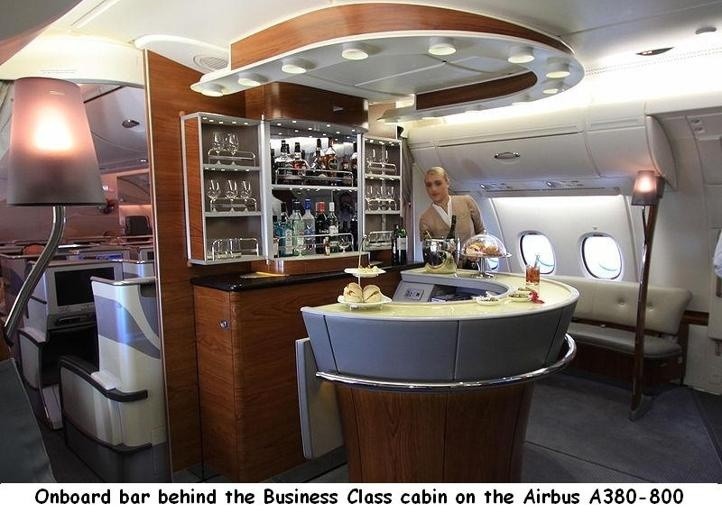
542, 82, 564, 96
509, 46, 537, 65
340, 42, 371, 62
544, 61, 571, 79
199, 83, 225, 99
627, 170, 660, 247
235, 70, 266, 88
281, 56, 307, 75
0, 74, 113, 348
426, 36, 457, 57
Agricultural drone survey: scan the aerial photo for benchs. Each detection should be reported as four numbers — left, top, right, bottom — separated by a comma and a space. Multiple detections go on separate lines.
543, 269, 694, 364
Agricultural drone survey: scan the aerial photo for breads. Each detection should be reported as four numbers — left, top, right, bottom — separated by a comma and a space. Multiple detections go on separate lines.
363, 284, 381, 302
344, 282, 364, 302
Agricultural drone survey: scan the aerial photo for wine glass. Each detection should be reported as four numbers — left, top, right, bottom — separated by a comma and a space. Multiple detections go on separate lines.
207, 130, 252, 259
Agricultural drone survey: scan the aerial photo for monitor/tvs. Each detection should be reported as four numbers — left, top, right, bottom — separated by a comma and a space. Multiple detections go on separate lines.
0, 235, 155, 330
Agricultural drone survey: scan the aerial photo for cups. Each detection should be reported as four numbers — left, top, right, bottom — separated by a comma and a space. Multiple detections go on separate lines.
526, 264, 540, 285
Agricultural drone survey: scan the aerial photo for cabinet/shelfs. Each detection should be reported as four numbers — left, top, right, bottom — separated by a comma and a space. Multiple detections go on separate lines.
364, 136, 406, 252
179, 107, 264, 267
242, 117, 372, 276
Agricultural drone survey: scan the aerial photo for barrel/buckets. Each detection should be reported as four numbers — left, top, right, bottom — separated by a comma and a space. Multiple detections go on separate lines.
422, 237, 461, 273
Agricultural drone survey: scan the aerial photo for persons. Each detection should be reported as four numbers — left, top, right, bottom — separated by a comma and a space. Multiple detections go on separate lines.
419, 166, 485, 270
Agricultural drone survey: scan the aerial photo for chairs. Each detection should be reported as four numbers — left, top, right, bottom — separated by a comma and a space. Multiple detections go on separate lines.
21, 242, 46, 256
52, 273, 169, 448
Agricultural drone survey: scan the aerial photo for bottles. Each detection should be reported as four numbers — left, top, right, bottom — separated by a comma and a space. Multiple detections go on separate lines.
366, 149, 407, 267
446, 214, 456, 240
270, 137, 358, 258
423, 229, 432, 240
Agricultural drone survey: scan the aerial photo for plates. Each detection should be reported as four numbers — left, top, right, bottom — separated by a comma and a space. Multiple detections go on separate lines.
338, 294, 392, 309
478, 291, 531, 304
344, 268, 387, 278
